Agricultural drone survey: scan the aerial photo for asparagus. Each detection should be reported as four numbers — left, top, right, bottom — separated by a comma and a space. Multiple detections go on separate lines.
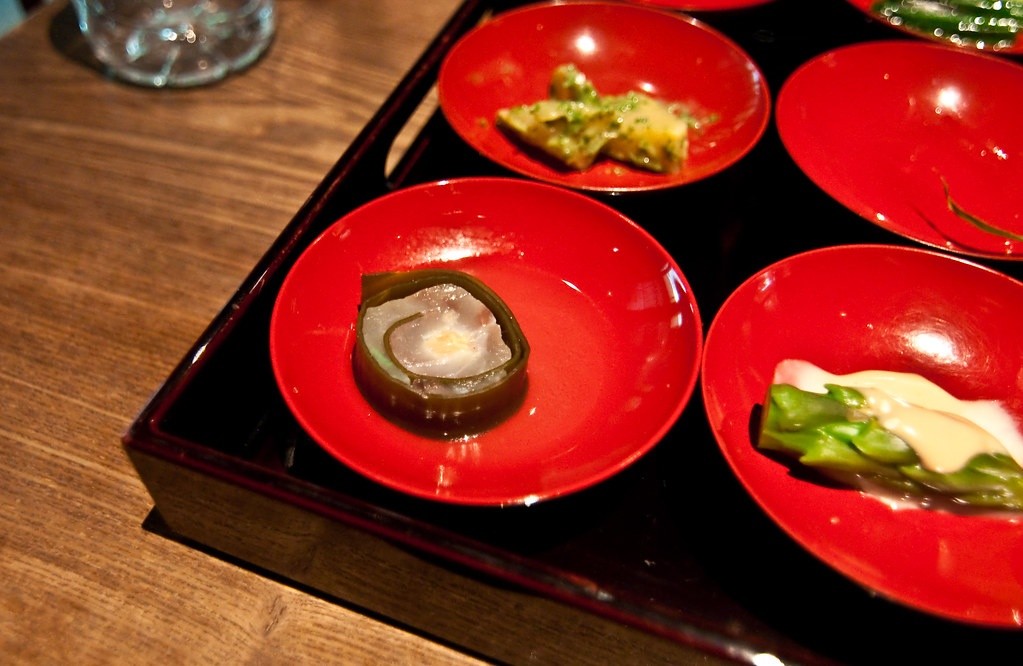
872, 0, 1023, 45
759, 384, 1023, 511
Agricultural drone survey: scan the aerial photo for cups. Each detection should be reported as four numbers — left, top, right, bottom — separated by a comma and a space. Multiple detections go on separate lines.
73, 1, 275, 88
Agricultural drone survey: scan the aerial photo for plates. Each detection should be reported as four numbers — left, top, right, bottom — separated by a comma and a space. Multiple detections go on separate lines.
269, 175, 703, 506
701, 244, 1023, 629
439, 1, 770, 193
850, 1, 1023, 56
628, 1, 769, 11
776, 41, 1023, 262
